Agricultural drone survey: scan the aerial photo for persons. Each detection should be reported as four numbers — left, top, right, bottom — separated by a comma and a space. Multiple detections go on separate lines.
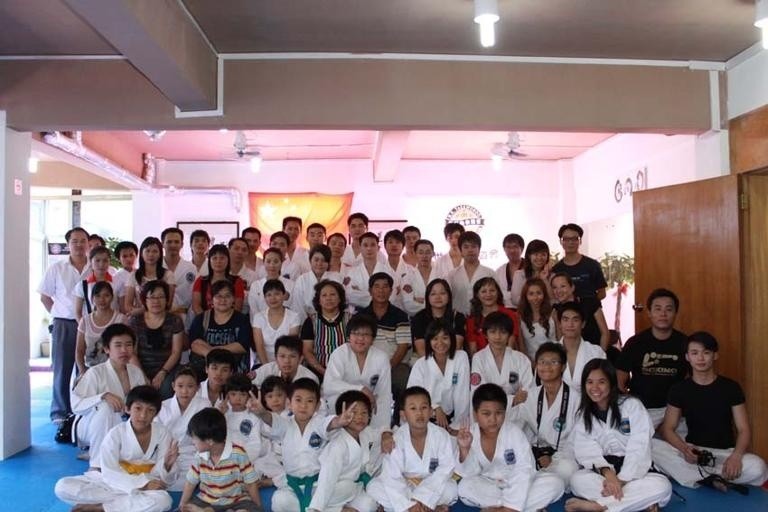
494, 233, 526, 294
305, 389, 396, 510
400, 226, 422, 269
189, 281, 251, 388
191, 244, 245, 314
348, 232, 400, 310
384, 229, 412, 317
155, 370, 209, 492
281, 217, 312, 277
613, 287, 693, 444
71, 281, 128, 461
553, 301, 607, 391
186, 230, 210, 278
113, 241, 139, 282
128, 280, 184, 400
510, 240, 558, 310
125, 237, 177, 316
465, 278, 520, 365
551, 223, 607, 301
226, 238, 257, 313
254, 230, 300, 282
468, 310, 535, 428
36, 227, 94, 444
319, 312, 391, 475
365, 385, 458, 512
295, 244, 345, 316
457, 383, 561, 512
246, 335, 322, 391
260, 376, 292, 465
652, 330, 765, 492
410, 278, 465, 369
302, 280, 349, 384
250, 247, 295, 320
551, 272, 620, 359
444, 231, 508, 321
217, 374, 270, 468
404, 320, 470, 450
192, 349, 236, 409
242, 227, 267, 281
400, 239, 453, 320
160, 228, 197, 316
252, 278, 301, 370
520, 340, 580, 492
514, 277, 561, 376
74, 247, 127, 324
305, 223, 326, 254
55, 385, 182, 512
338, 213, 386, 269
87, 234, 118, 276
435, 223, 465, 276
246, 378, 357, 512
565, 358, 672, 512
352, 272, 411, 424
177, 407, 264, 512
325, 233, 354, 299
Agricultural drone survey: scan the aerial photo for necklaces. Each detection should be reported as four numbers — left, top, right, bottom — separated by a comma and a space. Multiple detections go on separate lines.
322, 310, 339, 320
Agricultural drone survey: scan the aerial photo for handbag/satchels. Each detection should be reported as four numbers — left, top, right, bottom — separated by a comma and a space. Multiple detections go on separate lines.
54, 413, 73, 445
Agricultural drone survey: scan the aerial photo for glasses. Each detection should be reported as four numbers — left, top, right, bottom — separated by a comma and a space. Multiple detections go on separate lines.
537, 360, 562, 366
351, 331, 373, 337
561, 237, 581, 242
146, 295, 165, 299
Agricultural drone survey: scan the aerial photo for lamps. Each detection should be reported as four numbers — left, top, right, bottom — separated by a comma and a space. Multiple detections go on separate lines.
754, 0, 768, 50
474, 0, 500, 48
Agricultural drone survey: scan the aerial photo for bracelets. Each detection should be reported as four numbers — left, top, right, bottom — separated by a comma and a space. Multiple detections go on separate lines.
161, 367, 170, 374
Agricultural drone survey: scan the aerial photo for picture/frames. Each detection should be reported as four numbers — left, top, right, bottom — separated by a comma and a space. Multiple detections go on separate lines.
177, 221, 239, 250
348, 219, 407, 247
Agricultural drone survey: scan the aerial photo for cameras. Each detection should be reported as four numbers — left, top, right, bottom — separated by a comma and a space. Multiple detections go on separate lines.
531, 446, 555, 470
692, 449, 713, 466
